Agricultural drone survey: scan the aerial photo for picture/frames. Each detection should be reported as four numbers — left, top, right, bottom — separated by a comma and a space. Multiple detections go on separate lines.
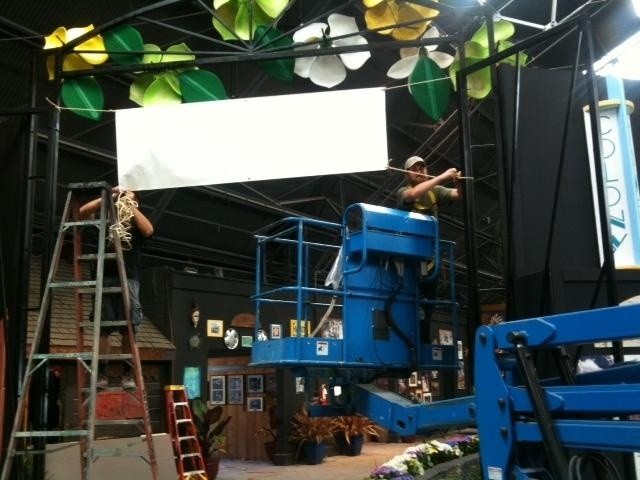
247, 373, 265, 395
227, 374, 244, 406
246, 398, 264, 413
210, 374, 227, 405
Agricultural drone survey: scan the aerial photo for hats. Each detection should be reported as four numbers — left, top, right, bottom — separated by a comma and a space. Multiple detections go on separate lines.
405, 156, 426, 171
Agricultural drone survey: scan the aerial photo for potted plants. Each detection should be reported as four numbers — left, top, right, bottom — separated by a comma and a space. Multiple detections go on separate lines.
186, 397, 233, 480
287, 409, 351, 465
329, 416, 384, 456
247, 425, 277, 463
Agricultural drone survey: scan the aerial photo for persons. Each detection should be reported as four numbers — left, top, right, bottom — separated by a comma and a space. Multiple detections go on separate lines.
396, 155, 464, 343
77, 183, 154, 392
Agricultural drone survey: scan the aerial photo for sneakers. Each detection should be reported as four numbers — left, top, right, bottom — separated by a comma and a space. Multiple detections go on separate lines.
97, 375, 108, 389
121, 373, 136, 390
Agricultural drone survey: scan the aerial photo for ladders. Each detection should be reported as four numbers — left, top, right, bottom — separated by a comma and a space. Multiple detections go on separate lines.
164, 385, 208, 479
0, 181, 161, 480
